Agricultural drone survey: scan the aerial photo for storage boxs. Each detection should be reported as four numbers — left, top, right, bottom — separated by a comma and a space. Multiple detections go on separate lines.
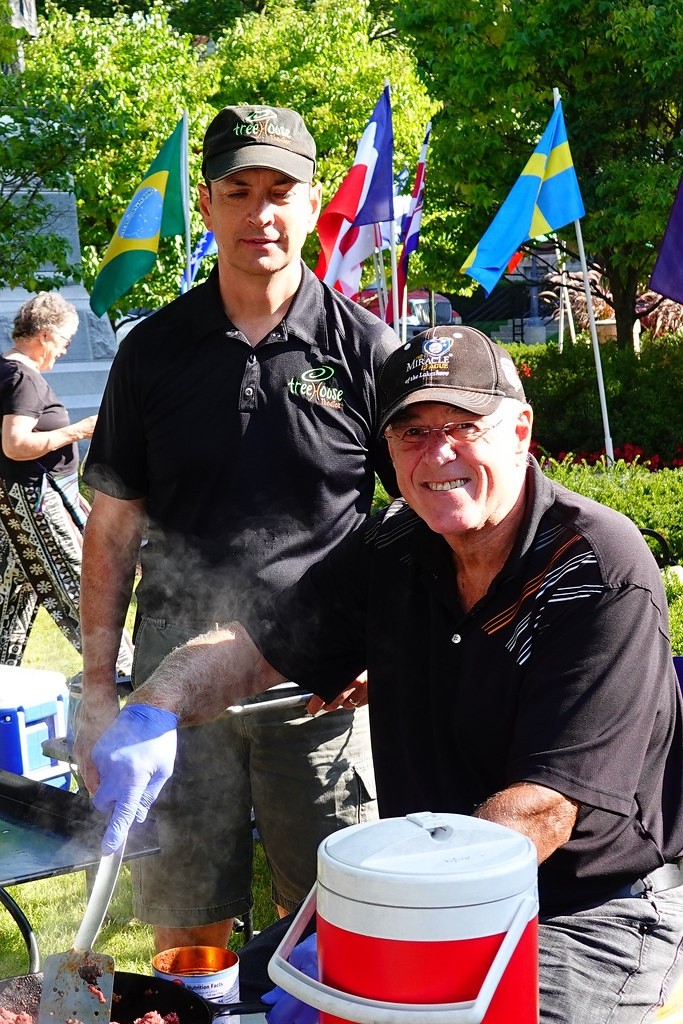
0, 663, 73, 782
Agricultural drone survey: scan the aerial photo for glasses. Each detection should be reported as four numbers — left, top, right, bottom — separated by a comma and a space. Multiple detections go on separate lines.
389, 420, 501, 449
55, 331, 70, 349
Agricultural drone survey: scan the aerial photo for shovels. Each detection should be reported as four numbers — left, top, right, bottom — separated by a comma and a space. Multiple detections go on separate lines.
37, 828, 129, 1024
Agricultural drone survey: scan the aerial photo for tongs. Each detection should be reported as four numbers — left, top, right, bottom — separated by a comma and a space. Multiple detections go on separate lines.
216, 681, 316, 719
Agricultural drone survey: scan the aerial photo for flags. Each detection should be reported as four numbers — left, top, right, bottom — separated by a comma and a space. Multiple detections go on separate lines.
89, 114, 191, 317
457, 96, 586, 300
310, 82, 432, 312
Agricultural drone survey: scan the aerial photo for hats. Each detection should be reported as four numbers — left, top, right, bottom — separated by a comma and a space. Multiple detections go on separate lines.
377, 324, 526, 434
202, 104, 314, 183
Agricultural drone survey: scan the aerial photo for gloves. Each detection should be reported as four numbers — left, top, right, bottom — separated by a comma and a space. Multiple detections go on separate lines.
261, 932, 319, 1024
93, 704, 176, 856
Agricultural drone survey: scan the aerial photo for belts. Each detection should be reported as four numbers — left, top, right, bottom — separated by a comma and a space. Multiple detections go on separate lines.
628, 862, 682, 900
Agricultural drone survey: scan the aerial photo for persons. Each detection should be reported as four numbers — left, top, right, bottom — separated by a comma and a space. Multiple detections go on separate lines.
92, 324, 683, 1024
0, 291, 131, 677
68, 103, 412, 970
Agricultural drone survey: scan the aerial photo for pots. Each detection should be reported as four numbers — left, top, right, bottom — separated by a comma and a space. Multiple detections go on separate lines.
1, 970, 270, 1024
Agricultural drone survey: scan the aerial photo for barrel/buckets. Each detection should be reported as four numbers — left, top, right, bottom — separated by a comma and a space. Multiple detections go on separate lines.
268, 813, 538, 1024
594, 320, 642, 360
152, 945, 241, 1024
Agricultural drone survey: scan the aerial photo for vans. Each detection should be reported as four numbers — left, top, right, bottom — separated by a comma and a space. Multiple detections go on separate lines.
349, 276, 461, 340
